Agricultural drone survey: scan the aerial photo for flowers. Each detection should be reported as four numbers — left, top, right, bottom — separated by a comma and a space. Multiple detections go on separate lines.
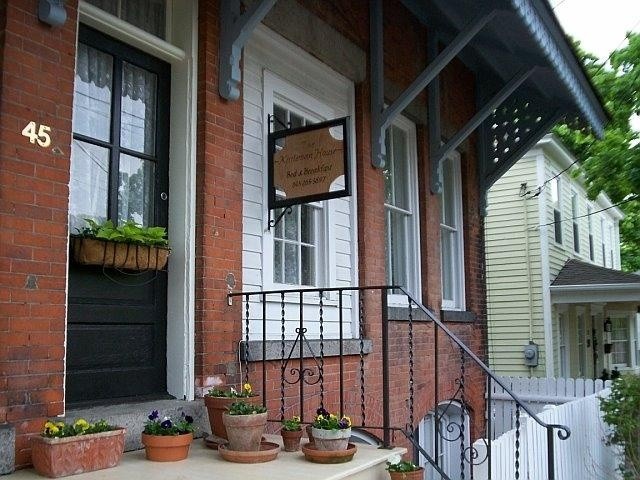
208, 383, 256, 400
144, 406, 195, 434
283, 415, 301, 431
311, 404, 351, 430
42, 415, 111, 438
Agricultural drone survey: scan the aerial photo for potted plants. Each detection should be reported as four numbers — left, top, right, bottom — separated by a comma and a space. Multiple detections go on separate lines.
385, 459, 425, 480
222, 402, 269, 451
71, 219, 172, 271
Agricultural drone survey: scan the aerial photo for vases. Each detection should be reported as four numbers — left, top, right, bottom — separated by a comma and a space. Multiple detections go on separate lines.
141, 433, 194, 462
311, 426, 352, 451
281, 429, 305, 452
29, 430, 126, 478
201, 395, 264, 443
